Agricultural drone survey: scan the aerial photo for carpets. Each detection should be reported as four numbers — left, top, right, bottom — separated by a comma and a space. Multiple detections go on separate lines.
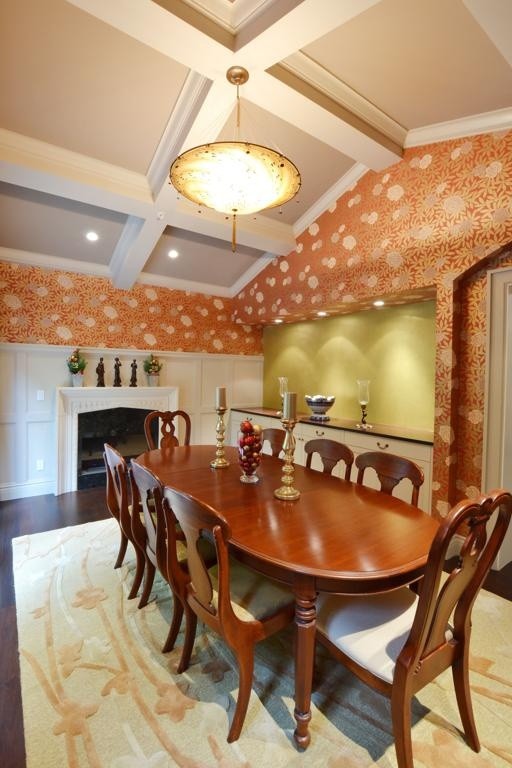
12, 517, 510, 768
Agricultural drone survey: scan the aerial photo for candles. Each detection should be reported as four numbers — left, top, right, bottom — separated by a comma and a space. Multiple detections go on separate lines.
215, 386, 227, 409
281, 391, 297, 417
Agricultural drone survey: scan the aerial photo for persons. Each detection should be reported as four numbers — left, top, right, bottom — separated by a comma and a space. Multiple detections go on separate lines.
111, 357, 121, 387
95, 357, 105, 387
128, 359, 139, 387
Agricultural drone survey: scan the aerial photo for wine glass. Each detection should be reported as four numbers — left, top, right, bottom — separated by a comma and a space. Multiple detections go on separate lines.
305, 394, 336, 421
236, 430, 264, 484
355, 379, 373, 429
276, 376, 289, 415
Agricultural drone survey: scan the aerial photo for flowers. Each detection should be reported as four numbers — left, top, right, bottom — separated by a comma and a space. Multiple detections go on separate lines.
142, 353, 165, 374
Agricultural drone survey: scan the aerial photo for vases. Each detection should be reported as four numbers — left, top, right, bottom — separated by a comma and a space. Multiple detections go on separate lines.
72, 374, 85, 389
66, 348, 88, 374
148, 372, 161, 387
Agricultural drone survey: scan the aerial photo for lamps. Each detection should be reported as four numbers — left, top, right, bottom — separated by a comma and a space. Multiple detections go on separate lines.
168, 67, 303, 254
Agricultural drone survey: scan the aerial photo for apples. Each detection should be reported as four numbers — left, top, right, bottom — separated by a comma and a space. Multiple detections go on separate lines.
238, 421, 263, 476
